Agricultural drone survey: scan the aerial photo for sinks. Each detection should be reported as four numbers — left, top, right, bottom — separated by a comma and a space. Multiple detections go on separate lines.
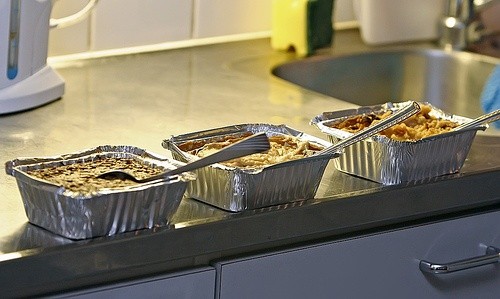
259, 41, 500, 136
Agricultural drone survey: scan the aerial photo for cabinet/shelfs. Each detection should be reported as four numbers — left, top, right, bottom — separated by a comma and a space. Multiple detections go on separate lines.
42, 207, 500, 299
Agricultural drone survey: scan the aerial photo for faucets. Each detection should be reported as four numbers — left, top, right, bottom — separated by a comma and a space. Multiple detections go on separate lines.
438, 0, 472, 51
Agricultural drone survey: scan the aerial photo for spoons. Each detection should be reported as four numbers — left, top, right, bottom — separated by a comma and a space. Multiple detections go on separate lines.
90, 131, 273, 187
